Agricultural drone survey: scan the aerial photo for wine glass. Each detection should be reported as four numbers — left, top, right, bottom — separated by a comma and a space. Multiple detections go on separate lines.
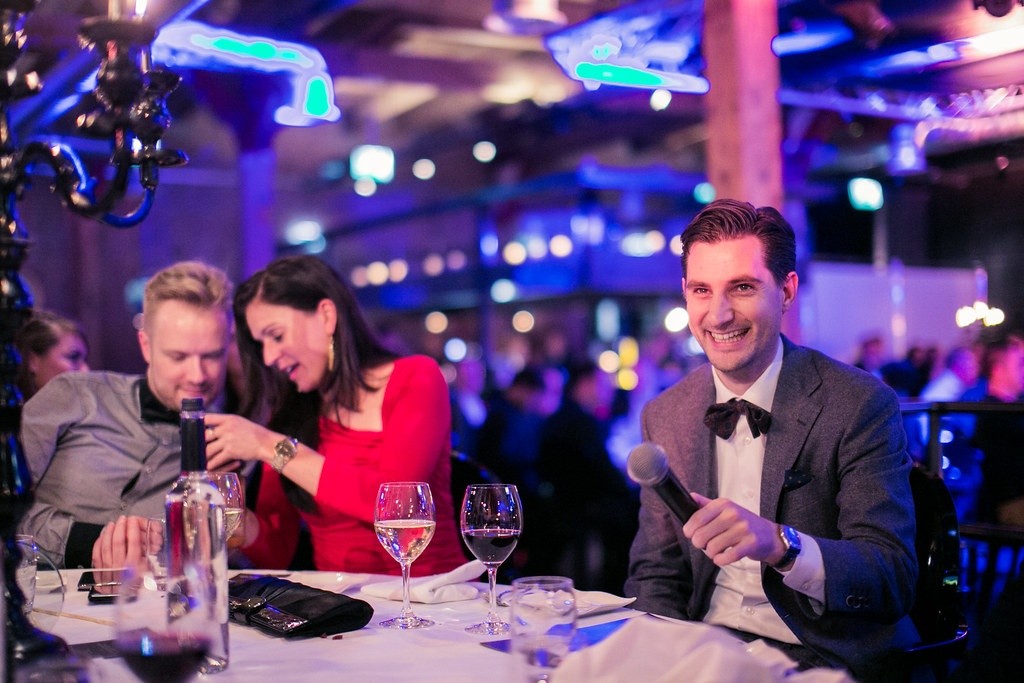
460, 484, 524, 637
209, 469, 246, 543
373, 482, 436, 629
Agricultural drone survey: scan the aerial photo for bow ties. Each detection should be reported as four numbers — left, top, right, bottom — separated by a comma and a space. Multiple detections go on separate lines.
703, 399, 773, 441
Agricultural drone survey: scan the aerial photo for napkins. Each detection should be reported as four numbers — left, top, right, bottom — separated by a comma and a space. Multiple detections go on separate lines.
573, 587, 637, 617
361, 559, 485, 602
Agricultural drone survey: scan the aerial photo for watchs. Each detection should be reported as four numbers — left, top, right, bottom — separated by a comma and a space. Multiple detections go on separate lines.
269, 436, 299, 476
769, 519, 802, 571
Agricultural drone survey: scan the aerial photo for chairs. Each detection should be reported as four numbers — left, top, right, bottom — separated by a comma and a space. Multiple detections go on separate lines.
451, 451, 529, 584
904, 462, 968, 683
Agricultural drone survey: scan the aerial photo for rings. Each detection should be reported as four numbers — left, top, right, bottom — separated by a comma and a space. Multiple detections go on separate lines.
208, 424, 217, 441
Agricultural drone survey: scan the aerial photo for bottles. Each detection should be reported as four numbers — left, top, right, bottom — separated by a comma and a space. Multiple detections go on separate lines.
163, 397, 231, 674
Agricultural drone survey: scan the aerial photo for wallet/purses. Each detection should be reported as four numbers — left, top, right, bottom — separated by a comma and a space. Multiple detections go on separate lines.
225, 571, 375, 640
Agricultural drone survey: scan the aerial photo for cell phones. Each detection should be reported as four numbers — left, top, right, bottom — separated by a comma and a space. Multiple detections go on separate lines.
77, 570, 132, 591
88, 583, 138, 604
178, 424, 246, 483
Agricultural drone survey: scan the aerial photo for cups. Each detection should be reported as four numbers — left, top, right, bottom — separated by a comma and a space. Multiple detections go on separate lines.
144, 513, 168, 579
509, 576, 579, 683
116, 573, 212, 683
15, 533, 37, 614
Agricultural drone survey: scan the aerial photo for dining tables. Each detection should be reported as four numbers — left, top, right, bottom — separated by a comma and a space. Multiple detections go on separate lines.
0, 570, 856, 683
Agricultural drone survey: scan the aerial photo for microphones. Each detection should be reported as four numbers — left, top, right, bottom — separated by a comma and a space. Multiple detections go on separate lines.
625, 442, 701, 526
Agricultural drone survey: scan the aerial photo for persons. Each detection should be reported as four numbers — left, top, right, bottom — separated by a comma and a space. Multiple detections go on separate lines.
20, 259, 236, 570
21, 308, 91, 399
843, 319, 1024, 528
373, 301, 707, 590
205, 254, 480, 584
606, 196, 920, 674
